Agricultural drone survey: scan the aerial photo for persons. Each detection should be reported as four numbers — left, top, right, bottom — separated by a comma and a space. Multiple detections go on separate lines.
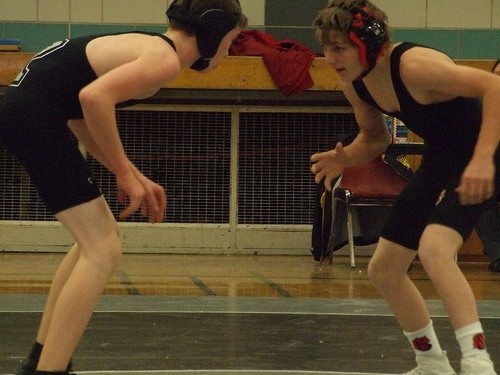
0, 0, 249, 375
473, 60, 500, 275
308, 0, 500, 375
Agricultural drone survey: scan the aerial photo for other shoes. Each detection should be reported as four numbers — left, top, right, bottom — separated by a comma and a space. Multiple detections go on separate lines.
396, 351, 458, 375
16, 360, 32, 375
454, 353, 496, 375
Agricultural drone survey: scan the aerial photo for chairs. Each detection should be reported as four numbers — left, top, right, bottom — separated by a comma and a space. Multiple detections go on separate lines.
329, 132, 418, 268
